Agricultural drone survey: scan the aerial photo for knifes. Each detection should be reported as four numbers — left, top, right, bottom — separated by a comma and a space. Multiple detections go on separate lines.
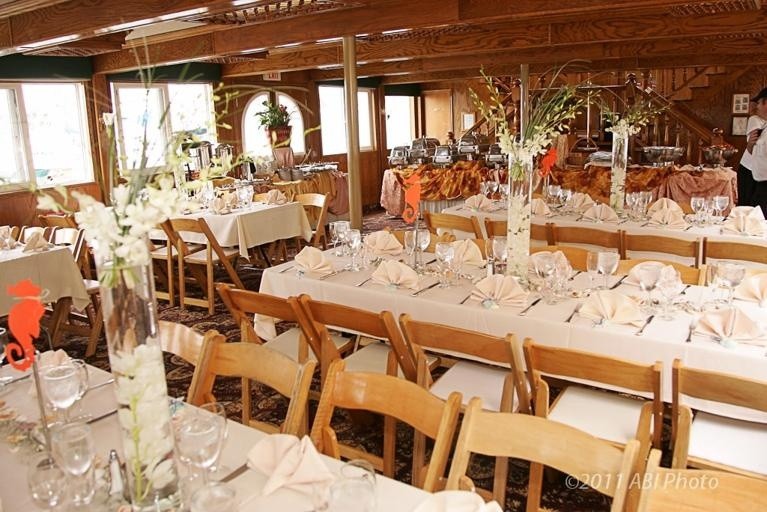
319, 268, 346, 280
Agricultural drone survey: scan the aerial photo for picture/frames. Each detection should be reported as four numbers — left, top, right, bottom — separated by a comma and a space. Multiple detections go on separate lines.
730, 116, 747, 136
731, 93, 750, 114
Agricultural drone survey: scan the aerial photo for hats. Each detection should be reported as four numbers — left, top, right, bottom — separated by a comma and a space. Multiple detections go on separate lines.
750, 87, 767, 102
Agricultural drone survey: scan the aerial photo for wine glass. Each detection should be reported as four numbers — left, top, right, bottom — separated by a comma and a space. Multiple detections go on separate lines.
136, 181, 253, 216
329, 219, 747, 321
476, 179, 729, 228
22, 358, 377, 511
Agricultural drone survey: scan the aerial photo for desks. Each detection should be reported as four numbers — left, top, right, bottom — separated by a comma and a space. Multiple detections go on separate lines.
380, 165, 737, 224
274, 172, 348, 228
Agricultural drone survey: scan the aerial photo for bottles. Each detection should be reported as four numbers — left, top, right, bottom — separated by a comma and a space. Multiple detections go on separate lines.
106, 449, 125, 498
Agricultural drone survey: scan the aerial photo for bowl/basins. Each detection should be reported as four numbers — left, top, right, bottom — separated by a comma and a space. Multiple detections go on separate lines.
701, 145, 739, 164
641, 146, 687, 163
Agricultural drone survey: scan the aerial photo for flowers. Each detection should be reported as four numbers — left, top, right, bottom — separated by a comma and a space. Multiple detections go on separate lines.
255, 101, 299, 129
468, 58, 604, 266
583, 81, 672, 205
32, 30, 236, 504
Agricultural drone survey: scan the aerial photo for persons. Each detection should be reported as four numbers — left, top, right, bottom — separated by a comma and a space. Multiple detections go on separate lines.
735, 101, 766, 207
746, 87, 766, 218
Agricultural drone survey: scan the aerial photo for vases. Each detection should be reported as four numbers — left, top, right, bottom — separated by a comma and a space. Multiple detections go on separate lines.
265, 126, 292, 148
610, 135, 628, 210
508, 155, 532, 277
